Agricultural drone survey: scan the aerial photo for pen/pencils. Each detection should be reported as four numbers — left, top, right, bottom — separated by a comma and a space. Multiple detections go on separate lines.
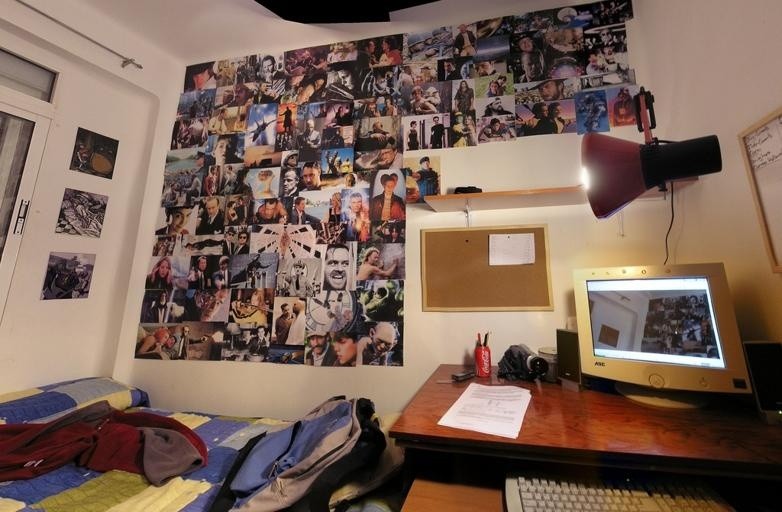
477, 333, 487, 346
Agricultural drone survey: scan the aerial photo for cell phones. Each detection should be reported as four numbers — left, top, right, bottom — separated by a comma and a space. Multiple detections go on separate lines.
452, 368, 476, 381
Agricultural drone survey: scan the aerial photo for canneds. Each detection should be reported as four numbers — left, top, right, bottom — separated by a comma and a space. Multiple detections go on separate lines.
474, 346, 491, 378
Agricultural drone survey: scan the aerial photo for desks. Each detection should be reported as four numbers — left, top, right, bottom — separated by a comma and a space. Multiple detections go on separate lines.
388, 365, 780, 511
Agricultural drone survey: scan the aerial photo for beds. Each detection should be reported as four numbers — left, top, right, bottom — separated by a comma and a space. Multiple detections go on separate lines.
0, 374, 403, 511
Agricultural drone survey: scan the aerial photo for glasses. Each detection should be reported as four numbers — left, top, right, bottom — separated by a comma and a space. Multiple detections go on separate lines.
240, 236, 246, 239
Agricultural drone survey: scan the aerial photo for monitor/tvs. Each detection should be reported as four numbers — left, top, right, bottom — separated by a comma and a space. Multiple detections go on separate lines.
573, 262, 753, 410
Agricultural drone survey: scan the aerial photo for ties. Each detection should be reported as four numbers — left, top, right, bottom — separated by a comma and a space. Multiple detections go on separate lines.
296, 274, 299, 290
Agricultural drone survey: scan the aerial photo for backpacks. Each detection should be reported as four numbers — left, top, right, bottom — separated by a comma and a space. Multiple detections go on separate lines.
210, 396, 386, 512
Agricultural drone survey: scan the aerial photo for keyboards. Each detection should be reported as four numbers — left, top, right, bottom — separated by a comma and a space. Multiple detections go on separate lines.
505, 464, 737, 512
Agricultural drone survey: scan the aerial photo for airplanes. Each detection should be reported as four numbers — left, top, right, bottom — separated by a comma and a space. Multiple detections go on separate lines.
250, 115, 277, 142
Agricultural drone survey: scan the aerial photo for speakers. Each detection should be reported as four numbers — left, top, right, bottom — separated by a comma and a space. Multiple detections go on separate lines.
743, 337, 782, 425
557, 328, 593, 393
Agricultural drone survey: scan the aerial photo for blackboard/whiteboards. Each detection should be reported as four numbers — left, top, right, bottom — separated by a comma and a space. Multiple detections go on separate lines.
737, 106, 782, 273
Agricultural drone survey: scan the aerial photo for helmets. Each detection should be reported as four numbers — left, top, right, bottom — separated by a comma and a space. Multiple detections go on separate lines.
408, 28, 444, 48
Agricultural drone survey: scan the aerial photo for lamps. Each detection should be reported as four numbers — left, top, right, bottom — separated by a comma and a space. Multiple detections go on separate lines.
579, 85, 724, 221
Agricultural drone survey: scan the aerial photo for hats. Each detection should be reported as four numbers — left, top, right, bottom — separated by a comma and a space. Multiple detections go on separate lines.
527, 78, 567, 90
382, 173, 398, 186
282, 150, 299, 168
219, 255, 231, 266
226, 226, 237, 234
420, 157, 429, 166
454, 112, 464, 117
293, 260, 303, 268
306, 331, 329, 338
